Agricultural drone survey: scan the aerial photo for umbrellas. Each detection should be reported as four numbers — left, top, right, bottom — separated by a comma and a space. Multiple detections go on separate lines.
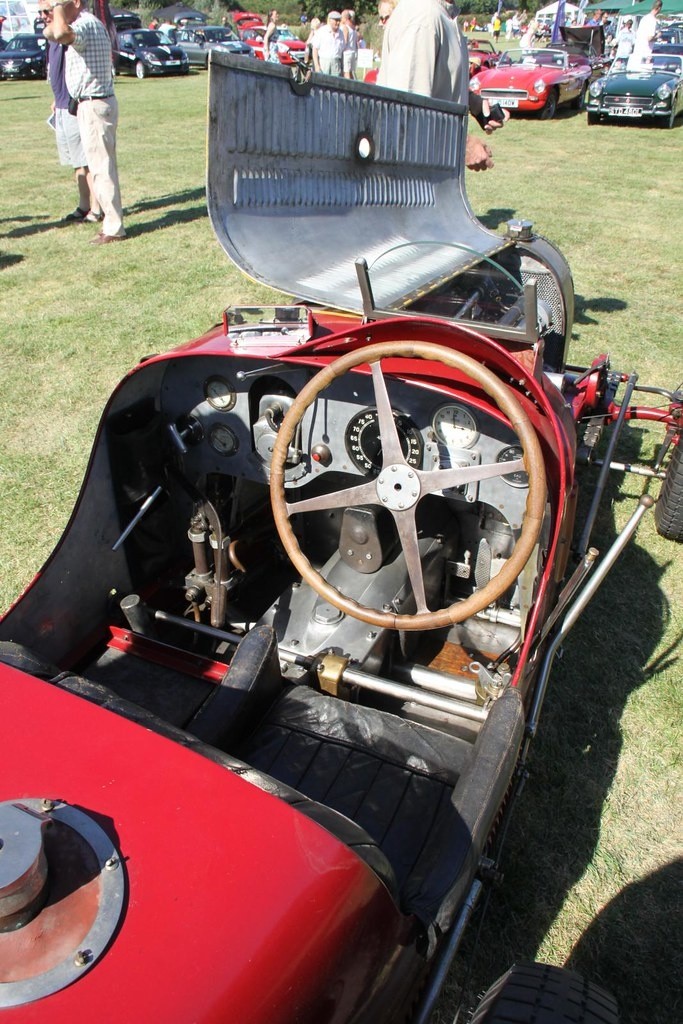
148, 1, 214, 23
108, 8, 141, 19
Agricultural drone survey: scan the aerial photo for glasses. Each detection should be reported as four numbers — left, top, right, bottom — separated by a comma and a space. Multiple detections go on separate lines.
37, 6, 54, 16
380, 15, 390, 20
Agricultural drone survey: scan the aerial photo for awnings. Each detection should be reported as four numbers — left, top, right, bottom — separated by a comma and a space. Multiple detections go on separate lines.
534, 0, 588, 35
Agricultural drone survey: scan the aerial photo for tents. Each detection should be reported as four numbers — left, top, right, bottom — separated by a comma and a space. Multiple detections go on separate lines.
582, 0, 683, 38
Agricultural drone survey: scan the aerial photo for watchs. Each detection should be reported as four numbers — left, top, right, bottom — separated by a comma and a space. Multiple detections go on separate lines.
50, 1, 64, 10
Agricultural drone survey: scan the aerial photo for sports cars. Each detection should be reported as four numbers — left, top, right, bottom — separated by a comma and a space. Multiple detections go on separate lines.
468, 47, 593, 121
584, 20, 683, 129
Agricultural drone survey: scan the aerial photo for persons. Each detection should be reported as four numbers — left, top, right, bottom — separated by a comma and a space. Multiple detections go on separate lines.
584, 8, 603, 26
609, 19, 634, 61
39, 0, 130, 246
263, 1, 510, 172
570, 20, 578, 27
148, 19, 191, 44
463, 9, 551, 63
220, 17, 234, 41
598, 11, 615, 60
634, 1, 664, 69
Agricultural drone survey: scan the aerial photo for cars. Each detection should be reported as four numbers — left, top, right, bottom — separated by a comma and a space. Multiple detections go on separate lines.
228, 9, 307, 65
172, 25, 256, 71
117, 28, 191, 79
0, 32, 49, 81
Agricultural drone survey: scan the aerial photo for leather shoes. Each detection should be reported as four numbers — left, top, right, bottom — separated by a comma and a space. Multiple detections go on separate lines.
90, 230, 127, 245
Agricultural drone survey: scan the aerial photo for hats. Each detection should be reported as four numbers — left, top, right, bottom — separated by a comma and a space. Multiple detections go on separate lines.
327, 11, 342, 19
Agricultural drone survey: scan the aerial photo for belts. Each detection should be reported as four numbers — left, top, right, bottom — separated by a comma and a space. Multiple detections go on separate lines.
76, 93, 114, 102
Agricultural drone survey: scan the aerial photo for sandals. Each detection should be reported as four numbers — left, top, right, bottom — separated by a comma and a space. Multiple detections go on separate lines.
65, 207, 105, 223
